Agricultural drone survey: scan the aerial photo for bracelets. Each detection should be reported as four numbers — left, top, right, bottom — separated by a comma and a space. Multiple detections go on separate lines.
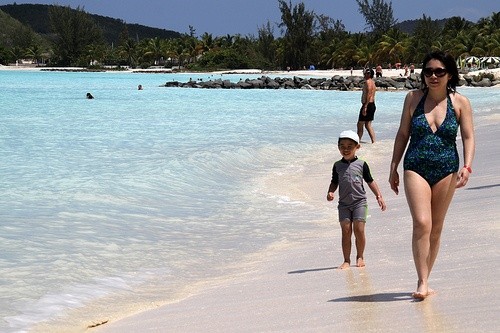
464, 166, 472, 173
376, 196, 382, 199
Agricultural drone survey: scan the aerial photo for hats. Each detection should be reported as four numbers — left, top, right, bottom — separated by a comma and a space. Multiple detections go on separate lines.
340, 131, 359, 142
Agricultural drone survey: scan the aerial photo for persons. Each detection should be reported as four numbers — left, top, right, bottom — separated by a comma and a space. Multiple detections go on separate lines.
189, 65, 306, 83
87, 93, 94, 99
327, 130, 386, 269
388, 51, 476, 300
138, 85, 143, 90
351, 62, 415, 77
357, 69, 376, 144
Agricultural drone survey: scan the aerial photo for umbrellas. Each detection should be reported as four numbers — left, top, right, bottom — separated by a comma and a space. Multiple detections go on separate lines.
461, 56, 500, 64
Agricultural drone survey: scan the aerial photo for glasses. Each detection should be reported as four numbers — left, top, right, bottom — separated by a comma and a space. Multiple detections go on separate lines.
423, 68, 448, 77
